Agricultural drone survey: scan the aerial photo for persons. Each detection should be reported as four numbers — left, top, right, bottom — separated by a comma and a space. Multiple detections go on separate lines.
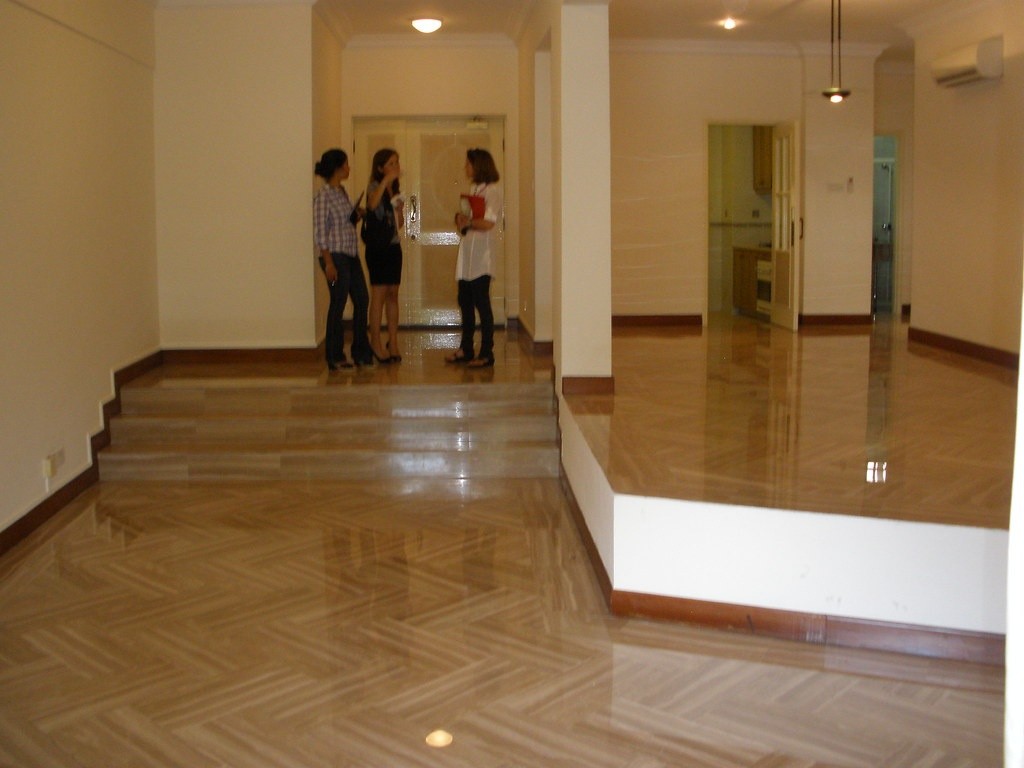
365, 149, 404, 364
446, 148, 501, 369
313, 148, 374, 370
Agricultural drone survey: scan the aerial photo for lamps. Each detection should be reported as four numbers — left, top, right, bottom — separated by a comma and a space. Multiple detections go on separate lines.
821, 0, 851, 103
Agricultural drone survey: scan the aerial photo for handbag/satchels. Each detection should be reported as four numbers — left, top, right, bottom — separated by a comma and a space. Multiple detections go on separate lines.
360, 206, 395, 247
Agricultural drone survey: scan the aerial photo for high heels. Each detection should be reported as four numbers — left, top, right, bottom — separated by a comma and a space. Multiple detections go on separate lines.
386, 340, 402, 363
370, 346, 394, 365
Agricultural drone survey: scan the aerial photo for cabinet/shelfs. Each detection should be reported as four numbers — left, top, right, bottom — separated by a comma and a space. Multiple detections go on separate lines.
752, 126, 772, 194
733, 246, 772, 322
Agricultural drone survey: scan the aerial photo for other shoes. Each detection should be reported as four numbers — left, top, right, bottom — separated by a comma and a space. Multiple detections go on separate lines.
353, 356, 373, 365
467, 357, 495, 368
444, 350, 475, 363
328, 357, 354, 369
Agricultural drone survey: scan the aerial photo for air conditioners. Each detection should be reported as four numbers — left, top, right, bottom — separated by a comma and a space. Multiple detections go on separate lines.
931, 36, 1004, 87
